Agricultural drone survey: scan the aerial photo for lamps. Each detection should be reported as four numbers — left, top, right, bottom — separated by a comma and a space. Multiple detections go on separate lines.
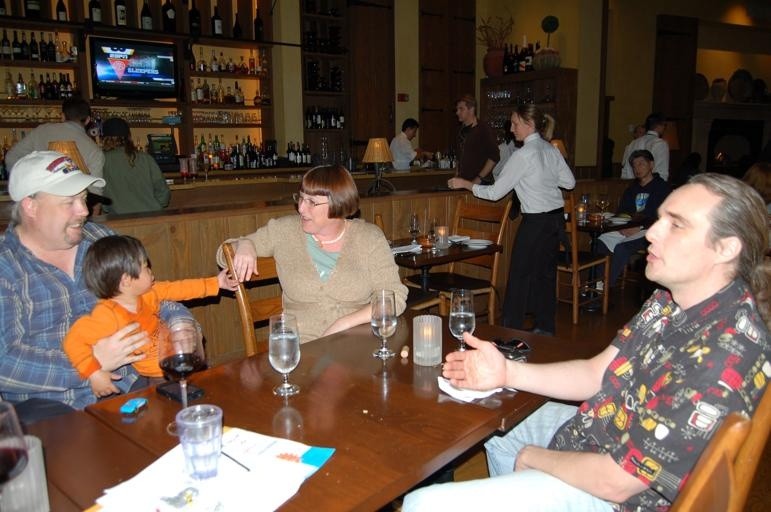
361, 137, 397, 195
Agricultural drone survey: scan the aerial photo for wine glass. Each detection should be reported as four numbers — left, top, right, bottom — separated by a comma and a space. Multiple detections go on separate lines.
371, 359, 399, 399
0, 402, 29, 511
271, 398, 306, 443
369, 289, 397, 358
268, 311, 303, 397
409, 214, 420, 245
153, 316, 206, 436
449, 289, 476, 350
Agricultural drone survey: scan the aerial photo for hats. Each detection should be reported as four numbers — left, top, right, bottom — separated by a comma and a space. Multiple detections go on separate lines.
9, 149, 106, 200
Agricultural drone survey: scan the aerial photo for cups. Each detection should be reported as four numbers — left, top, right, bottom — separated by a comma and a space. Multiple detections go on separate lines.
434, 226, 449, 250
175, 402, 224, 479
412, 365, 442, 398
412, 314, 442, 367
0, 435, 50, 512
428, 217, 440, 241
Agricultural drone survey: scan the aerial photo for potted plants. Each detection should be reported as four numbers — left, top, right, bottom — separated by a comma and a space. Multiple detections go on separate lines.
532, 15, 561, 71
475, 4, 514, 77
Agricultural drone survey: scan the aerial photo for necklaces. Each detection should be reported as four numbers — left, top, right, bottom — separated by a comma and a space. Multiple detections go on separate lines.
312, 217, 347, 246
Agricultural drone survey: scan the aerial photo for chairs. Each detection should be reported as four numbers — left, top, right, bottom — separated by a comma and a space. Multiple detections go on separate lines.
222, 242, 283, 356
667, 382, 771, 512
555, 190, 611, 325
616, 248, 646, 289
375, 214, 447, 317
401, 196, 512, 325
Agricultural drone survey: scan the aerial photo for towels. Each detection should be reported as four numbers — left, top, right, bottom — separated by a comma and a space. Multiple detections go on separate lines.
596, 212, 616, 220
588, 228, 647, 253
448, 234, 471, 244
391, 244, 423, 255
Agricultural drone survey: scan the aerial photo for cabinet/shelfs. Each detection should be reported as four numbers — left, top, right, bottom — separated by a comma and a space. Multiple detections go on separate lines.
479, 67, 577, 179
299, 0, 351, 159
654, 14, 771, 170
0, 0, 275, 169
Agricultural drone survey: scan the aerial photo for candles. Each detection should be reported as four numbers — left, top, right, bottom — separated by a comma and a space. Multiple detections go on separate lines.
578, 207, 584, 225
438, 228, 448, 246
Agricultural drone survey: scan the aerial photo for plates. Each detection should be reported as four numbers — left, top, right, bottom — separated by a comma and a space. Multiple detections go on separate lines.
464, 238, 494, 248
574, 193, 633, 225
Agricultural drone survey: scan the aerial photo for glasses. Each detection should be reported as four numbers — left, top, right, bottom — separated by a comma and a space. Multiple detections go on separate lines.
291, 190, 330, 208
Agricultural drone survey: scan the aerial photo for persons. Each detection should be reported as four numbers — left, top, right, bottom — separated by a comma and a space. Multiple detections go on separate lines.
414, 170, 771, 511
216, 162, 411, 345
389, 65, 771, 203
0, 149, 209, 431
65, 234, 239, 396
5, 97, 106, 216
591, 149, 674, 291
99, 118, 173, 217
450, 100, 577, 336
471, 114, 566, 186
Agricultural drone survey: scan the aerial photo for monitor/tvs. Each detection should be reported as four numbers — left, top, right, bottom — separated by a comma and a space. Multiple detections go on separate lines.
89, 35, 181, 96
147, 133, 177, 160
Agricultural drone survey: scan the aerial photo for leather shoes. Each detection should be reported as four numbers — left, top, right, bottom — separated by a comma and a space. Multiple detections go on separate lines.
526, 327, 556, 338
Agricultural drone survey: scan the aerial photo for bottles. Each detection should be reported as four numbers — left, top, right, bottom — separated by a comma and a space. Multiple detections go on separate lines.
0, 1, 349, 185
435, 144, 460, 169
483, 40, 561, 147
